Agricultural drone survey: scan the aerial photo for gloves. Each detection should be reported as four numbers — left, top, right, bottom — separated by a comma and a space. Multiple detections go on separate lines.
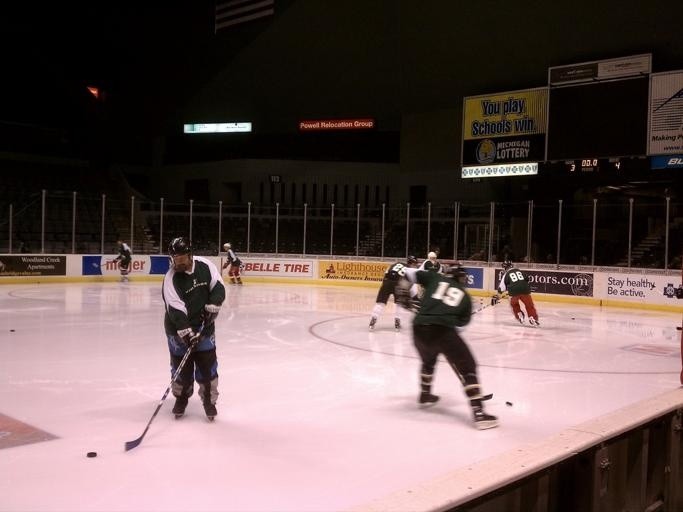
200, 304, 219, 328
177, 327, 201, 348
491, 295, 500, 306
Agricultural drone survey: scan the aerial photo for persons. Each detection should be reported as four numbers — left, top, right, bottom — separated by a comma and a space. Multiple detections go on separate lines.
162, 237, 226, 418
113, 240, 131, 282
369, 248, 540, 431
223, 243, 243, 284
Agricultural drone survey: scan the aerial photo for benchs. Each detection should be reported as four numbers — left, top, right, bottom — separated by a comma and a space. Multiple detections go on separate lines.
0, 201, 160, 255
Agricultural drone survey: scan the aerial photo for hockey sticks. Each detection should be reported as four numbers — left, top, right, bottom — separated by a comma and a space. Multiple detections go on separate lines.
93, 261, 113, 268
443, 354, 493, 402
125, 321, 204, 450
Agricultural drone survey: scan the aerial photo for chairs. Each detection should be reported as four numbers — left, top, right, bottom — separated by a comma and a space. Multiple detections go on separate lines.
367, 220, 483, 260
148, 216, 370, 256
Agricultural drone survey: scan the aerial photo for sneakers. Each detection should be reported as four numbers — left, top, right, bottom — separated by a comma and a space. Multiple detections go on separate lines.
203, 405, 217, 417
474, 414, 497, 421
395, 318, 400, 326
369, 317, 376, 325
420, 394, 439, 403
172, 398, 189, 414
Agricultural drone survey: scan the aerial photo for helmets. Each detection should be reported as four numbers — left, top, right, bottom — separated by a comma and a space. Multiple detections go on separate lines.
224, 243, 231, 249
428, 252, 437, 264
444, 263, 467, 287
502, 260, 513, 270
168, 237, 193, 272
407, 256, 417, 266
